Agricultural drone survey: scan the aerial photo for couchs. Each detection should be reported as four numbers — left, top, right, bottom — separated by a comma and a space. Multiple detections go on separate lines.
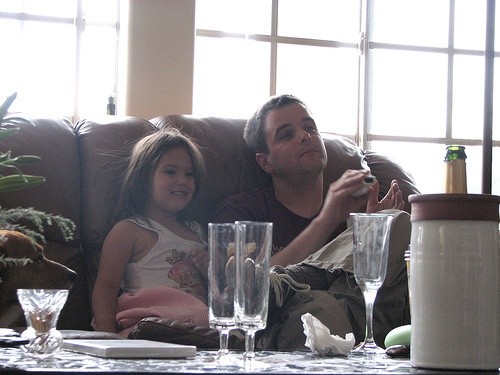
0, 114, 424, 348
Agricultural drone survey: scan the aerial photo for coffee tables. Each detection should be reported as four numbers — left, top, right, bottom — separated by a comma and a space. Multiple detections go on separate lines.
0, 348, 500, 375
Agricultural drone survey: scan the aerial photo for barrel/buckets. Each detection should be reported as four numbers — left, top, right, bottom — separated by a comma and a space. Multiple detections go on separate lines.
409, 194, 500, 370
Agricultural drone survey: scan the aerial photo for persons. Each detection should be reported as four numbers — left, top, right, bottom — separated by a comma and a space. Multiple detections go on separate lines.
218, 93, 412, 351
90, 126, 226, 341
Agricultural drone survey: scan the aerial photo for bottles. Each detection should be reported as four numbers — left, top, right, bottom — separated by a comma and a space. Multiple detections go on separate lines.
444, 146, 467, 194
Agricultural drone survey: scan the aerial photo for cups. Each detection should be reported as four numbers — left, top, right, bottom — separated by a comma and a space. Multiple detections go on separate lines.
19, 289, 70, 362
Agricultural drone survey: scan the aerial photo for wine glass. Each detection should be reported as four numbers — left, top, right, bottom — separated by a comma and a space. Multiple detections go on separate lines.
234, 221, 273, 374
344, 212, 394, 365
202, 223, 246, 368
